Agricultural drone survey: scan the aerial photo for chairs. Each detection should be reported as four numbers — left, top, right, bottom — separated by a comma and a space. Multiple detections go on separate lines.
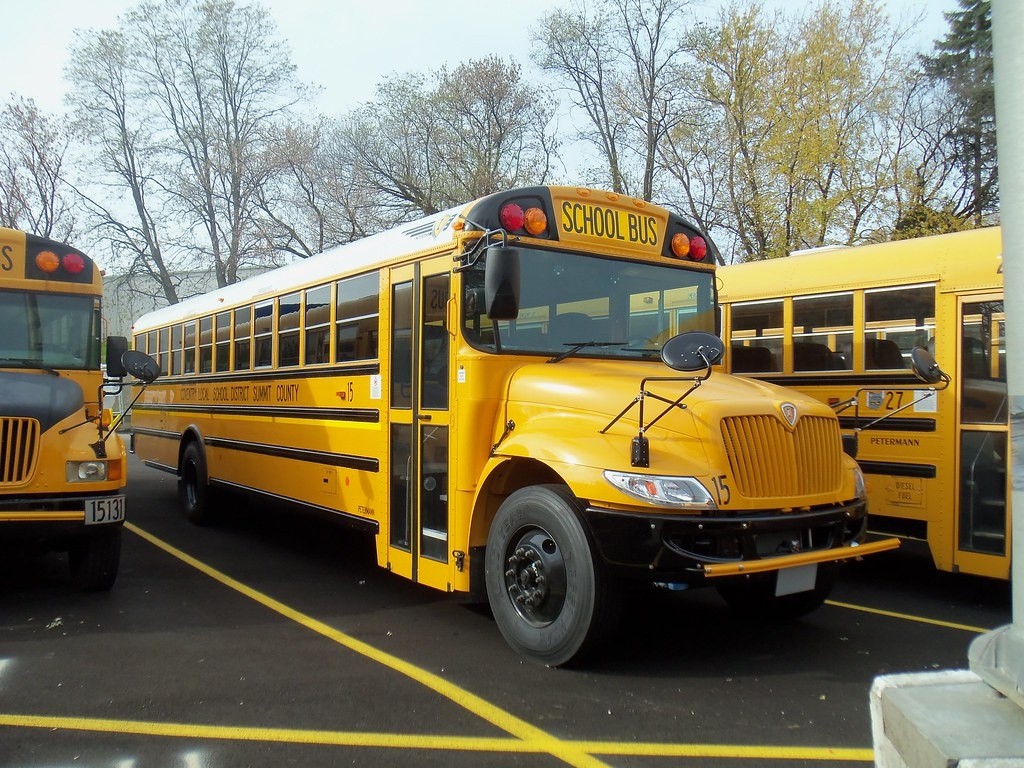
548, 311, 594, 350
850, 339, 907, 370
794, 342, 846, 371
961, 337, 1006, 409
719, 345, 771, 372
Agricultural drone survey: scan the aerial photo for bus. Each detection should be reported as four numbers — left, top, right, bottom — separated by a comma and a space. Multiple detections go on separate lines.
1, 224, 165, 595
127, 184, 904, 668
426, 224, 1011, 579
185, 276, 449, 491
